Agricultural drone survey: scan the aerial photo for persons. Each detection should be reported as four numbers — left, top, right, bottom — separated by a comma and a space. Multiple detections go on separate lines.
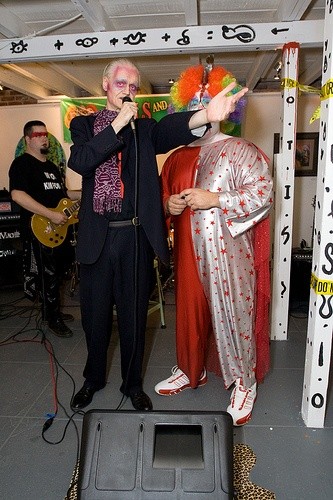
68, 58, 249, 412
154, 62, 274, 428
8, 120, 80, 337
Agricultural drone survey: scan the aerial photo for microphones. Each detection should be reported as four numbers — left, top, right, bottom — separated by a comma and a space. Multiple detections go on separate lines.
60, 162, 64, 175
123, 97, 136, 132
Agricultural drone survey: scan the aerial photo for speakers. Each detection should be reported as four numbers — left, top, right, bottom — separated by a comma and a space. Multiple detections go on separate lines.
0, 223, 22, 291
77, 409, 233, 500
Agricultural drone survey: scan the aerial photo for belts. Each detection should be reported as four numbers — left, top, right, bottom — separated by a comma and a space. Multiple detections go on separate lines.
107, 216, 141, 227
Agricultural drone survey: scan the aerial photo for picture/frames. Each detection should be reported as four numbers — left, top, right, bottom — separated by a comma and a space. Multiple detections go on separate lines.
272, 132, 319, 177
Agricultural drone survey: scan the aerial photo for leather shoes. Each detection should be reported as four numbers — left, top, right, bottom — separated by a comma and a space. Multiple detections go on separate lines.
71, 380, 107, 409
130, 390, 153, 410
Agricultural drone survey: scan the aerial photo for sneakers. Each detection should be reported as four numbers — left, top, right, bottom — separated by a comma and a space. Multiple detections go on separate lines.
154, 364, 207, 396
225, 377, 257, 426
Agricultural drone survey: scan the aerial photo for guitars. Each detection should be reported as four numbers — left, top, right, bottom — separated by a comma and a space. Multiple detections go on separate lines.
29, 197, 81, 250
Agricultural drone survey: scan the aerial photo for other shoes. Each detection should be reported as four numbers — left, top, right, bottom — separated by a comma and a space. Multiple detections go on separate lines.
44, 313, 74, 321
47, 316, 73, 338
